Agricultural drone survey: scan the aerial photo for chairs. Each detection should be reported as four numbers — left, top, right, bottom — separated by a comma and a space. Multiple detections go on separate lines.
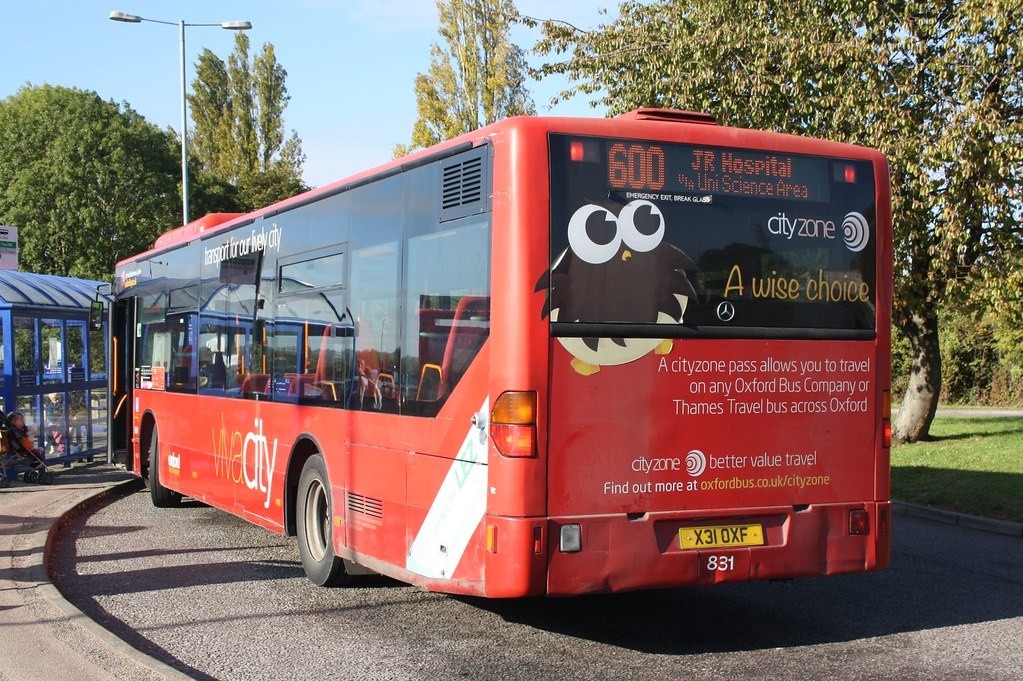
179, 295, 490, 403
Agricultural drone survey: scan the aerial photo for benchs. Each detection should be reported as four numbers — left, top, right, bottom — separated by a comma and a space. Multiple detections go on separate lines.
18, 405, 108, 432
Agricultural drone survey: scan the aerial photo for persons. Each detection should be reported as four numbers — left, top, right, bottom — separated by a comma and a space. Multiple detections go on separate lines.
5, 411, 43, 469
40, 393, 89, 457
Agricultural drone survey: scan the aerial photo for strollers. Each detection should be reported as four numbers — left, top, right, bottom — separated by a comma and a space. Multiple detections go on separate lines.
0, 409, 54, 489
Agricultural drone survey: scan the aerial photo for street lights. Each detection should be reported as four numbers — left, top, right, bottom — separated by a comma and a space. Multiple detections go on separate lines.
108, 10, 253, 225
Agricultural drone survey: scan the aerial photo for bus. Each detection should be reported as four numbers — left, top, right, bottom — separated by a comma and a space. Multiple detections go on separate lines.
87, 104, 897, 603
